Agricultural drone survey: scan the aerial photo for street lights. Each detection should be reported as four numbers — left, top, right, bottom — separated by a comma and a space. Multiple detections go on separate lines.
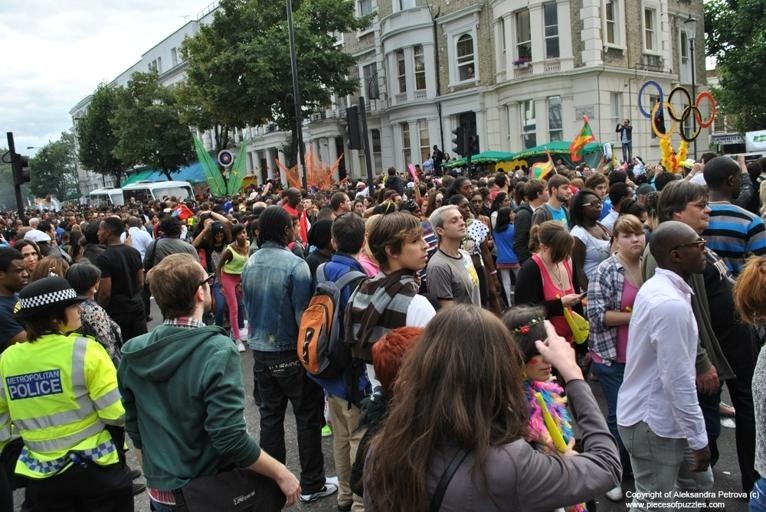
21, 145, 59, 154
681, 12, 699, 162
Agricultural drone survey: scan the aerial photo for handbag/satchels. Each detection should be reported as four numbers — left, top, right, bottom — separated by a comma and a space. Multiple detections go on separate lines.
174, 467, 287, 511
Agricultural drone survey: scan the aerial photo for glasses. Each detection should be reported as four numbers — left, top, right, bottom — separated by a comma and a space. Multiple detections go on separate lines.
202, 272, 215, 286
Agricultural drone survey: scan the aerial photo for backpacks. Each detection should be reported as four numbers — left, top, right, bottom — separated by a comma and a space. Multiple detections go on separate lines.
295, 261, 367, 378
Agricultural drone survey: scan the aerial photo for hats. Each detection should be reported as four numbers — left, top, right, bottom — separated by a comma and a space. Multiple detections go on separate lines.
18, 274, 89, 311
24, 229, 51, 243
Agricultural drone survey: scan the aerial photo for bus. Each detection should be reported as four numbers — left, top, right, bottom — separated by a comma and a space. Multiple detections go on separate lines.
122, 180, 196, 203
89, 186, 125, 206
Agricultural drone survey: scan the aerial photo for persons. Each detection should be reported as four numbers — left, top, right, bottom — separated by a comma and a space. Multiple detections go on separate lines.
0, 119, 766, 512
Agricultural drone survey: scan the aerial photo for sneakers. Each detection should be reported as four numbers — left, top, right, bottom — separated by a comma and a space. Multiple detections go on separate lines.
232, 325, 248, 352
719, 401, 735, 417
320, 424, 332, 438
299, 475, 338, 503
606, 486, 623, 501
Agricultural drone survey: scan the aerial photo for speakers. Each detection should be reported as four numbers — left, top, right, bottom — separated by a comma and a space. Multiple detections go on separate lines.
347, 104, 364, 150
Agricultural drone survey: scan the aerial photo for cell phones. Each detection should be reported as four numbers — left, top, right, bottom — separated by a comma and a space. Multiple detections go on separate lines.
580, 292, 587, 300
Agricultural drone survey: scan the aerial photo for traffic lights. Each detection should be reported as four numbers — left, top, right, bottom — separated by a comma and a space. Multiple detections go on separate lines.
451, 127, 466, 155
13, 153, 31, 184
342, 103, 362, 149
468, 135, 480, 155
